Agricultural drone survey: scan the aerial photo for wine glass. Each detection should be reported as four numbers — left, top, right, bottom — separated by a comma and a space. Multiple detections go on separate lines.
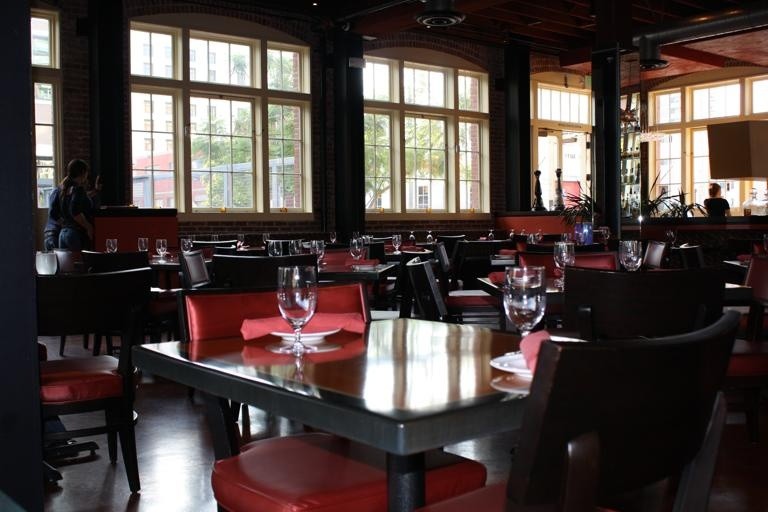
180, 226, 683, 272
275, 264, 321, 356
553, 242, 577, 285
503, 266, 548, 353
106, 238, 169, 263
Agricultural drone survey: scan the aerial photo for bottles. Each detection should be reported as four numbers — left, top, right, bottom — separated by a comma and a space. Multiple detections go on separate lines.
742, 186, 768, 216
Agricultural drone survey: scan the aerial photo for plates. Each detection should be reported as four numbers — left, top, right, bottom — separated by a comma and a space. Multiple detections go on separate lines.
487, 353, 533, 373
269, 328, 344, 343
269, 342, 341, 354
491, 374, 532, 393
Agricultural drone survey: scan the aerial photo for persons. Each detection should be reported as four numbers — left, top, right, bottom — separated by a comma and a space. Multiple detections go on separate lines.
704, 182, 731, 225
44, 176, 89, 252
58, 158, 103, 253
